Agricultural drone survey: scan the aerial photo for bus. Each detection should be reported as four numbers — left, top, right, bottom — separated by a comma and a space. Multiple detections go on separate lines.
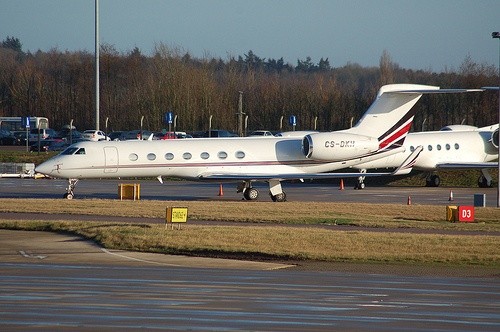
0, 117, 49, 146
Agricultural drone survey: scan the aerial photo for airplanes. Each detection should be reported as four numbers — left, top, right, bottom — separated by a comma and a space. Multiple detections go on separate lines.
276, 124, 500, 189
34, 82, 499, 202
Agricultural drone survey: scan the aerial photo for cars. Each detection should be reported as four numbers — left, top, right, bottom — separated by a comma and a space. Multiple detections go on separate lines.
28, 127, 274, 153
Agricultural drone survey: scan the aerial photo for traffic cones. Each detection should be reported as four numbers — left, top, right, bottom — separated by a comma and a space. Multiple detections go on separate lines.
340, 179, 345, 190
217, 184, 224, 196
406, 196, 411, 205
448, 189, 455, 202
